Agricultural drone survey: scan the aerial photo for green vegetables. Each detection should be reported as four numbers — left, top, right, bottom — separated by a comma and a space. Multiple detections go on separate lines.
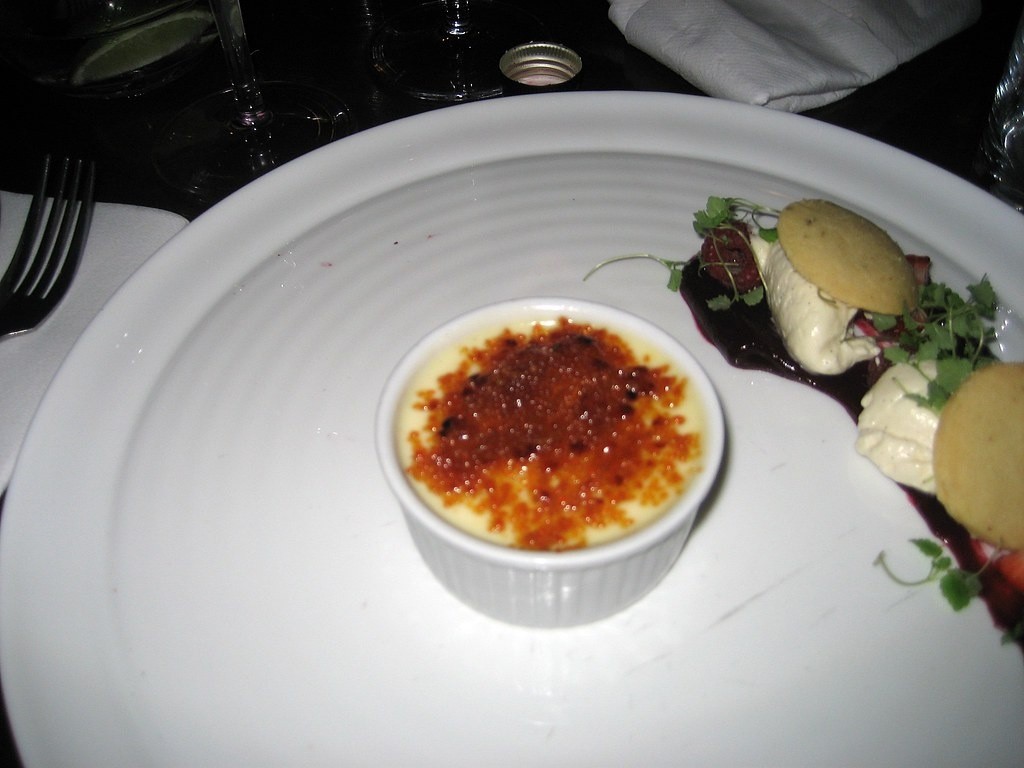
580, 196, 1024, 650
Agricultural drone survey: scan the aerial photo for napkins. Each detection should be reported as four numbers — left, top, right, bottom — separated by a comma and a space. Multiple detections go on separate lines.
607, 0, 983, 115
0, 189, 190, 496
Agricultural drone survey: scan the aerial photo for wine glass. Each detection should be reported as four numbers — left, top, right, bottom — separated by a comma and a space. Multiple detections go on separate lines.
146, 0, 352, 204
369, 0, 531, 102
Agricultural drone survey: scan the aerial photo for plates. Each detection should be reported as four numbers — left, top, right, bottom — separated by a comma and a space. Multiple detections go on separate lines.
0, 90, 1024, 767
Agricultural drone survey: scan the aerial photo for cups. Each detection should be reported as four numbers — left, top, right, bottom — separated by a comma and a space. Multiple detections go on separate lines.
376, 296, 729, 630
16, 0, 223, 100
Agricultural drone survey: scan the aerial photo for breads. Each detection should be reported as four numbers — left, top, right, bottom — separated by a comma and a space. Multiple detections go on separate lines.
776, 199, 918, 315
930, 360, 1024, 549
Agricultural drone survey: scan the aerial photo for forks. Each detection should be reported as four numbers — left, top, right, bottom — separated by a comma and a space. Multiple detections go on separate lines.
1, 153, 99, 345
